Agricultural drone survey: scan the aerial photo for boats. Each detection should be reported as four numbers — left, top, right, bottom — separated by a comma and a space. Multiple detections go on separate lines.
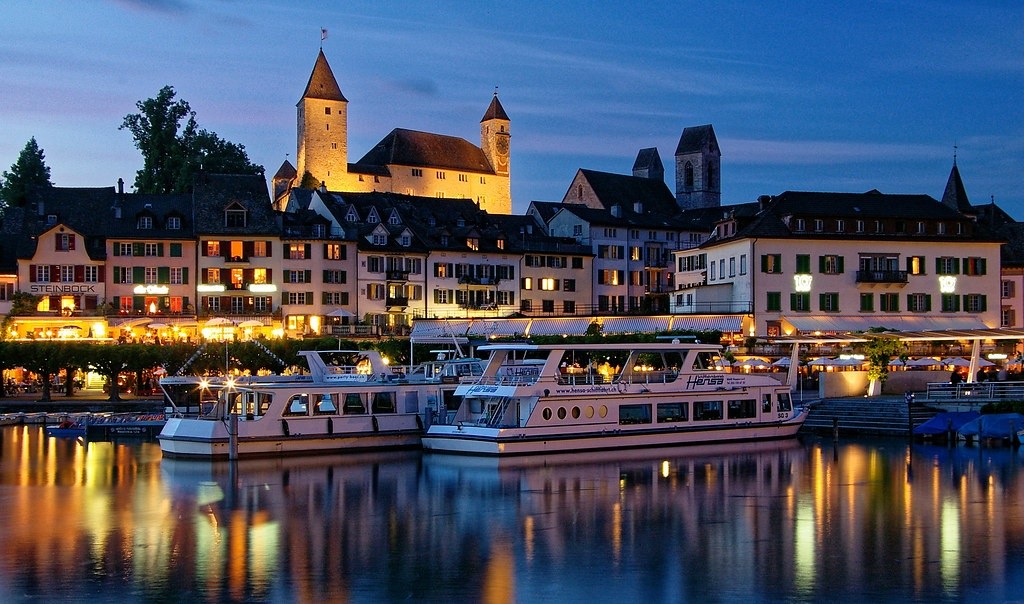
155, 349, 562, 457
419, 341, 814, 458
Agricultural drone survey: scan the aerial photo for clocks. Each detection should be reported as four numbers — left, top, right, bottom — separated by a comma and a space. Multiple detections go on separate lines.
495, 137, 510, 156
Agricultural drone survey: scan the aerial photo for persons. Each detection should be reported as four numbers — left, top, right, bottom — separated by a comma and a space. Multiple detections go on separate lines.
950, 369, 963, 398
976, 366, 987, 382
987, 360, 1024, 381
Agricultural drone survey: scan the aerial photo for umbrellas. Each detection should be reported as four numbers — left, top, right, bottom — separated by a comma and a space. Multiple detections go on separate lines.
324, 307, 356, 325
709, 355, 1024, 372
204, 317, 231, 328
238, 318, 264, 338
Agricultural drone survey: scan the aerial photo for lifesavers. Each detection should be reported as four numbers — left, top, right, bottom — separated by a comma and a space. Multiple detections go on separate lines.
618, 380, 628, 393
